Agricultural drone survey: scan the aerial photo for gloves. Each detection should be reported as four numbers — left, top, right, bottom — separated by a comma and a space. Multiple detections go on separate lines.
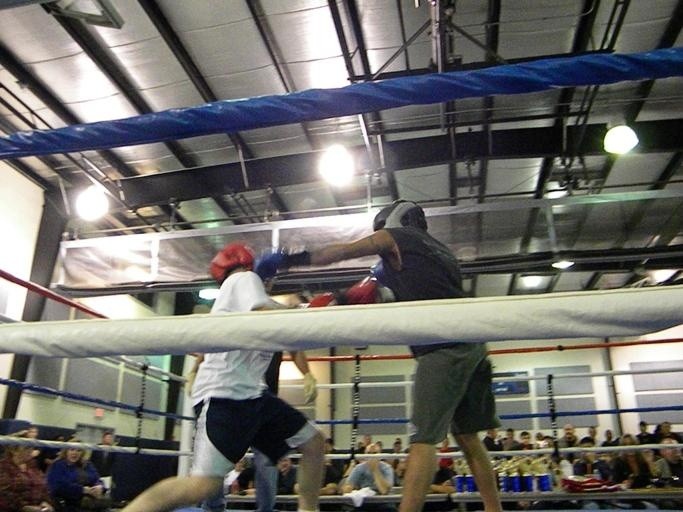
254, 249, 310, 281
370, 260, 386, 285
306, 293, 334, 307
343, 277, 376, 305
304, 372, 318, 407
184, 371, 196, 396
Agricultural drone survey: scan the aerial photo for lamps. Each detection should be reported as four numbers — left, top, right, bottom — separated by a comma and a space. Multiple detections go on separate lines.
316, 140, 357, 188
603, 115, 640, 156
74, 182, 111, 224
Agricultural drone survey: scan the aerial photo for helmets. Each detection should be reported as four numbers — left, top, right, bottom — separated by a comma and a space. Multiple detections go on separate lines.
373, 200, 427, 231
211, 244, 254, 285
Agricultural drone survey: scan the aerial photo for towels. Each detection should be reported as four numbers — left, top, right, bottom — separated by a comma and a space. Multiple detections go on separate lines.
342, 484, 379, 508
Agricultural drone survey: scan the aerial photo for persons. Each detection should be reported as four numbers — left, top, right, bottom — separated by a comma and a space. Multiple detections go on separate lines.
253, 198, 503, 512
118, 239, 326, 512
0, 426, 117, 511
430, 463, 457, 493
319, 435, 410, 495
439, 437, 454, 469
183, 276, 318, 511
224, 458, 254, 495
482, 421, 683, 510
277, 457, 296, 495
